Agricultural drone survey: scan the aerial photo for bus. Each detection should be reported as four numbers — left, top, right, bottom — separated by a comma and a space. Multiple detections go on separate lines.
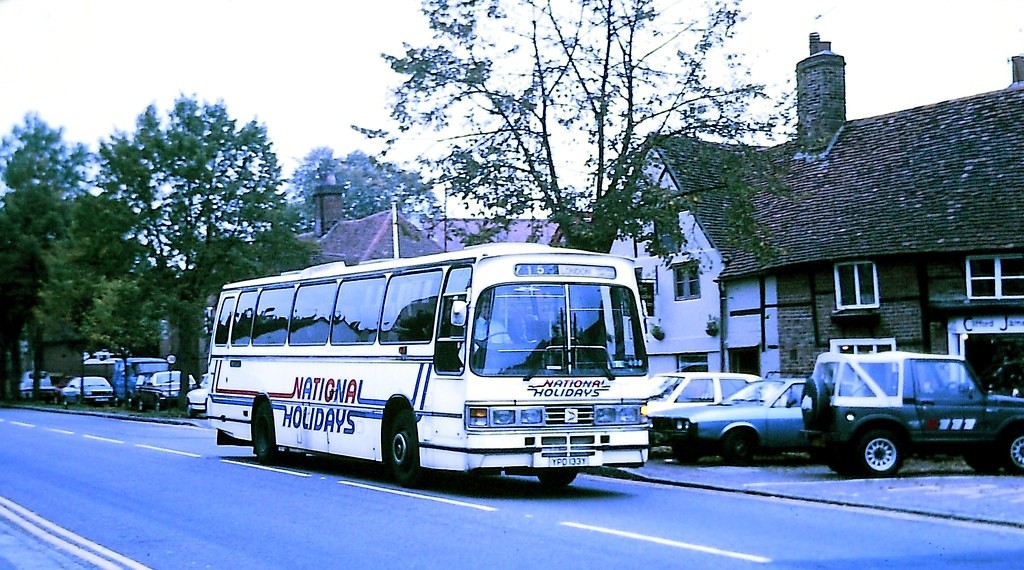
207, 240, 649, 488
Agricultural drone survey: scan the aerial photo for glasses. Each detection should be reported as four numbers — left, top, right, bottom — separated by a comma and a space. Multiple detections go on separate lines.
483, 306, 491, 308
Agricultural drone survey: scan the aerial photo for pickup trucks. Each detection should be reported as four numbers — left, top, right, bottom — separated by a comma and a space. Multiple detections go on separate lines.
21, 370, 59, 404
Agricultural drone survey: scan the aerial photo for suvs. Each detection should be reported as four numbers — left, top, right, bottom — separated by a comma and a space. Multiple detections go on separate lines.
800, 347, 1024, 476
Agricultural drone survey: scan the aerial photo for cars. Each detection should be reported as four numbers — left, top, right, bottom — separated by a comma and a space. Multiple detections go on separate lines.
61, 377, 114, 407
137, 371, 182, 414
650, 366, 818, 467
187, 373, 209, 418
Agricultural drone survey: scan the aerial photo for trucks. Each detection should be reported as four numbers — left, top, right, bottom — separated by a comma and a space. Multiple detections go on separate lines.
81, 357, 169, 409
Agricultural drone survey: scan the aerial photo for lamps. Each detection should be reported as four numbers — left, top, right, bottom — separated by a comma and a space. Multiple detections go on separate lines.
649, 320, 664, 341
706, 314, 719, 336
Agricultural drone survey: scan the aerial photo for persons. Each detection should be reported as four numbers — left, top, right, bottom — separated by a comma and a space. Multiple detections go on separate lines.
471, 298, 514, 358
